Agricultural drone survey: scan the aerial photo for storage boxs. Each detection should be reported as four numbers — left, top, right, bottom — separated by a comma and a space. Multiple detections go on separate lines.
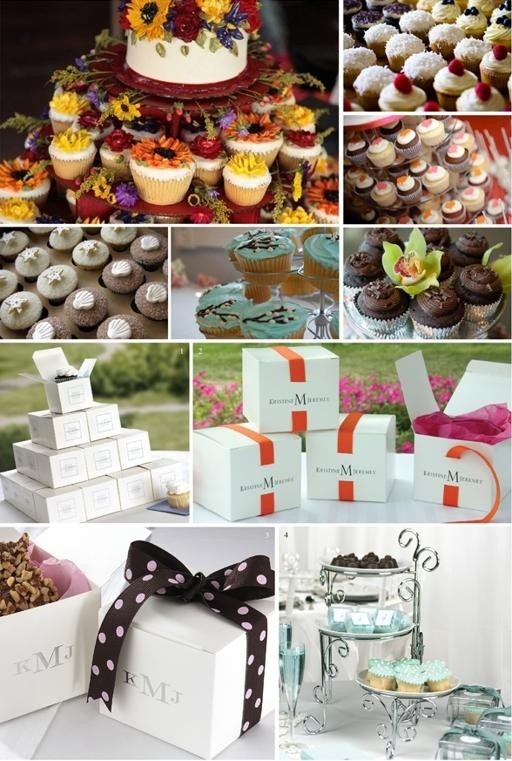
99, 594, 276, 760
0, 347, 183, 525
0, 525, 101, 727
194, 346, 512, 520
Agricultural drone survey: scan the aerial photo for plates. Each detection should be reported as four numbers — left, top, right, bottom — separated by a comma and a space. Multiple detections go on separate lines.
353, 668, 464, 700
343, 280, 509, 341
310, 615, 415, 643
316, 553, 415, 575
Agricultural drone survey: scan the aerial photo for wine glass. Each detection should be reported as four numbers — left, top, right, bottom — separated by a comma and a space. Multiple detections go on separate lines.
280, 641, 307, 752
279, 624, 305, 727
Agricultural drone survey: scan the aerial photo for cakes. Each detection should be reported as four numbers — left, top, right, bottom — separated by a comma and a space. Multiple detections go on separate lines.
0, 0, 339, 223
344, 115, 512, 224
344, 228, 503, 329
0, 227, 168, 339
368, 656, 449, 684
195, 227, 339, 339
343, 0, 512, 112
